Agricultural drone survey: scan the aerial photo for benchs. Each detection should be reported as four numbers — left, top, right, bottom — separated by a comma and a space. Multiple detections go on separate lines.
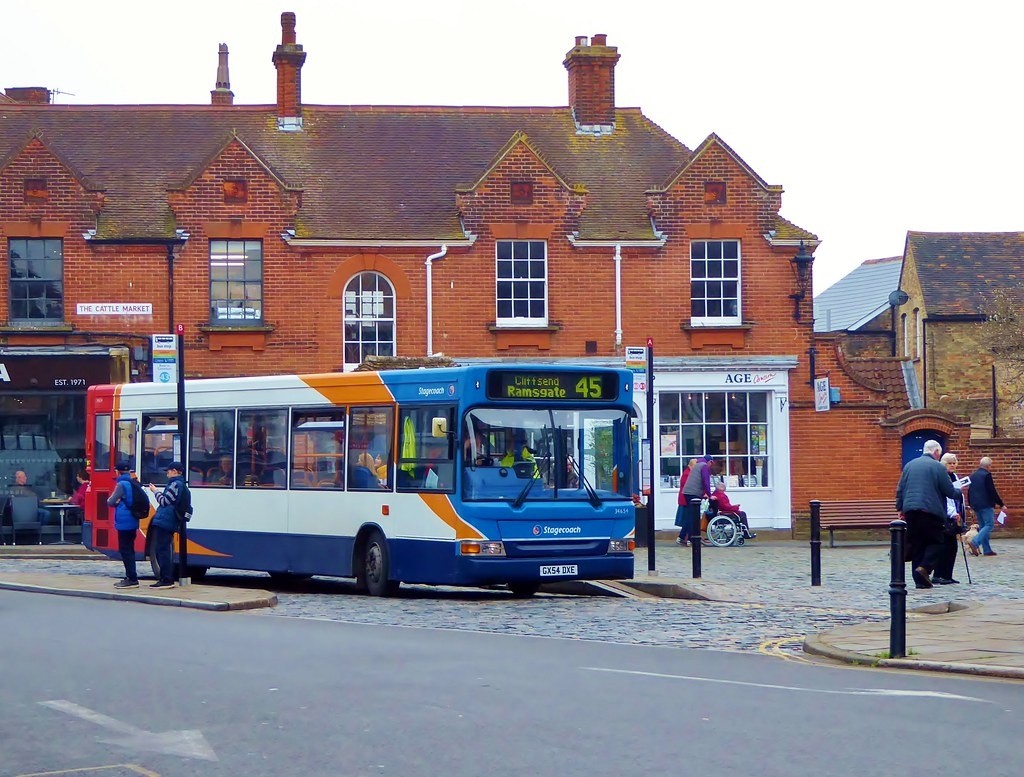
818, 499, 902, 549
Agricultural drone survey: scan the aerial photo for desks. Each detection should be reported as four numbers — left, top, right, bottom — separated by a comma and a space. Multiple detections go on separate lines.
43, 497, 80, 544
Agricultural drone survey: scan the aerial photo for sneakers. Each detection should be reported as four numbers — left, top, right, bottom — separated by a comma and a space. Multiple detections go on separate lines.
113, 577, 139, 589
150, 578, 175, 589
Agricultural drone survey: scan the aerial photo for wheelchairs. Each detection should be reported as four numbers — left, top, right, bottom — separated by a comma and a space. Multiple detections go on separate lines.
703, 497, 754, 548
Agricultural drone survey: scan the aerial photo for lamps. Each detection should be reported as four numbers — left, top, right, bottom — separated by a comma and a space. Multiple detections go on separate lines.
788, 240, 816, 320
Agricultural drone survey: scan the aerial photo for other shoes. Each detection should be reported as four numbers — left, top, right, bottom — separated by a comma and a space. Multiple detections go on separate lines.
984, 551, 996, 555
933, 577, 941, 583
968, 542, 978, 556
675, 536, 691, 547
742, 533, 756, 538
940, 578, 952, 584
914, 567, 933, 588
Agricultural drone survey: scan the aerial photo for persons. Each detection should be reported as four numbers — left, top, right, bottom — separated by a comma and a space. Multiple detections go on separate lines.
432, 430, 482, 488
894, 439, 966, 589
356, 452, 387, 485
711, 482, 756, 540
249, 453, 286, 485
15, 470, 51, 525
567, 456, 579, 488
674, 455, 718, 546
67, 470, 90, 525
106, 460, 140, 589
501, 431, 542, 479
968, 457, 1007, 557
210, 453, 247, 487
147, 461, 187, 588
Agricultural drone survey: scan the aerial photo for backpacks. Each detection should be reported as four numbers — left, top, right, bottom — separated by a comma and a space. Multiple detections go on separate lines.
168, 478, 193, 522
118, 478, 149, 519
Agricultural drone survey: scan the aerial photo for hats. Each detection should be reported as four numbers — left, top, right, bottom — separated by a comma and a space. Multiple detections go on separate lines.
330, 430, 344, 443
115, 461, 131, 471
704, 455, 715, 464
163, 462, 184, 472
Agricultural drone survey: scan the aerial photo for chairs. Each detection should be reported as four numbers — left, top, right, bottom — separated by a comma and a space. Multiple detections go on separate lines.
10, 496, 44, 545
146, 443, 382, 490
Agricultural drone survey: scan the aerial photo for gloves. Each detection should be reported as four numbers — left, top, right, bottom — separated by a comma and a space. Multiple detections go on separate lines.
953, 513, 962, 522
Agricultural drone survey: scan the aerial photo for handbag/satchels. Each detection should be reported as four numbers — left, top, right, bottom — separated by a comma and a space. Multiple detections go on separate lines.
996, 509, 1008, 527
898, 515, 913, 562
700, 510, 708, 532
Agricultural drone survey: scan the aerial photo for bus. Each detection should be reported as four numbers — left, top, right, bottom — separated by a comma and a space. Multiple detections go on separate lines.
82, 357, 650, 598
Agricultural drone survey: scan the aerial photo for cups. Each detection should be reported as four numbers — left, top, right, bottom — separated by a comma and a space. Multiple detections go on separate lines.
51, 492, 55, 497
